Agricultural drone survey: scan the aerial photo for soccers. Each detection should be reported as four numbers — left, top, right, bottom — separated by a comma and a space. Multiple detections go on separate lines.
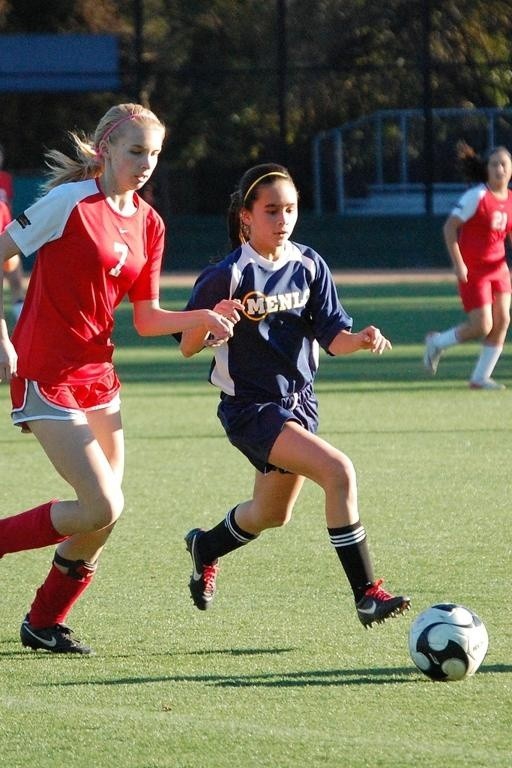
408, 603, 488, 683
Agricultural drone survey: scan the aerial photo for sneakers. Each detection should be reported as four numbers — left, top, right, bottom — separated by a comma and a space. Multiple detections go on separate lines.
354, 580, 410, 628
424, 332, 441, 373
185, 527, 219, 611
468, 376, 504, 391
20, 614, 91, 655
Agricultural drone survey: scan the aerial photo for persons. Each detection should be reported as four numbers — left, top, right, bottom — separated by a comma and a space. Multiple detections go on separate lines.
421, 145, 511, 391
0, 102, 235, 655
0, 147, 28, 324
169, 163, 413, 631
